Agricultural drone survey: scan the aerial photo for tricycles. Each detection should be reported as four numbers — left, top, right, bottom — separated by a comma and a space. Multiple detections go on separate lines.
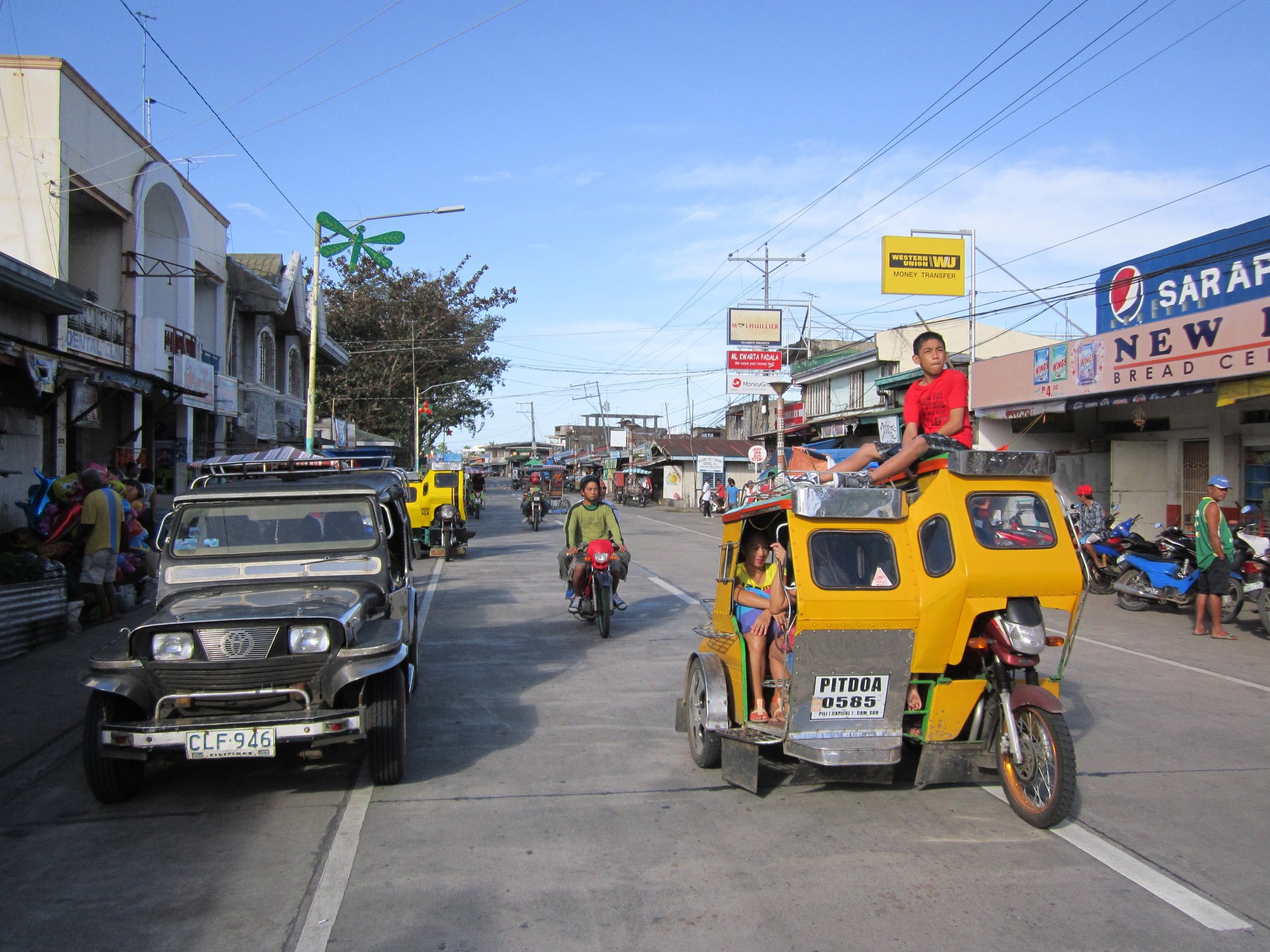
615, 468, 652, 507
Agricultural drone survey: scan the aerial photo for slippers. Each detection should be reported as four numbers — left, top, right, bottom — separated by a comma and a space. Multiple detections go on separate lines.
709, 516, 714, 518
1211, 633, 1237, 640
703, 516, 709, 518
1194, 629, 1210, 636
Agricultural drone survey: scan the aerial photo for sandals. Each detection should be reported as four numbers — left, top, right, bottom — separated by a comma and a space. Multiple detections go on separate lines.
749, 698, 770, 723
769, 697, 785, 717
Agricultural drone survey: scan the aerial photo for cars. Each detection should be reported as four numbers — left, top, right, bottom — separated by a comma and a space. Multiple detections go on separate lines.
487, 467, 501, 476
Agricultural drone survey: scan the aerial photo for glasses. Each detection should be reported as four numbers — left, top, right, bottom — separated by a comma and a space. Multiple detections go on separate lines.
600, 486, 607, 490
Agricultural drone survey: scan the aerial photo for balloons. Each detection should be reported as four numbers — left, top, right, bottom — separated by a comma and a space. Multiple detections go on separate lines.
17, 465, 148, 583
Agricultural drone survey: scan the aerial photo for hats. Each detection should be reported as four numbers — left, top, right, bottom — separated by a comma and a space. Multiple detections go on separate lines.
1207, 475, 1233, 490
748, 481, 752, 484
1073, 485, 1093, 495
706, 475, 713, 480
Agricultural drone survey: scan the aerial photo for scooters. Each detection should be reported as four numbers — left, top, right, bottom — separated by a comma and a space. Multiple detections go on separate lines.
698, 496, 727, 514
465, 488, 487, 520
989, 508, 1270, 639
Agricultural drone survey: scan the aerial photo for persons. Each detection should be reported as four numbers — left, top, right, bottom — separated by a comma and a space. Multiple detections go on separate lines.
72, 468, 123, 623
1193, 476, 1242, 641
108, 462, 158, 551
438, 454, 445, 462
521, 472, 551, 524
699, 471, 778, 520
784, 332, 972, 486
558, 475, 632, 613
1073, 483, 1106, 572
736, 529, 923, 736
470, 473, 486, 515
970, 494, 998, 547
637, 473, 653, 504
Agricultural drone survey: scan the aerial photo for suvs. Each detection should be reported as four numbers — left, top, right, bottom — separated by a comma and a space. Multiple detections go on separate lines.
80, 454, 422, 808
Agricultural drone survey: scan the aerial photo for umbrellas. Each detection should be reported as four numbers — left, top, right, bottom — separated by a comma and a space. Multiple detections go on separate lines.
524, 458, 544, 467
757, 467, 778, 480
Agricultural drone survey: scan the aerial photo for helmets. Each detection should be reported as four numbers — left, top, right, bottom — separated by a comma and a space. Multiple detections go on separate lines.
531, 472, 540, 484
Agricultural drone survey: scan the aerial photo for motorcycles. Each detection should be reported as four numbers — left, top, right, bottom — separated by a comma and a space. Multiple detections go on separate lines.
565, 539, 624, 639
523, 490, 550, 532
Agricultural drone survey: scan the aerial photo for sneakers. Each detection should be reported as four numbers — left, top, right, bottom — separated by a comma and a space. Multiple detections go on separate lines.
568, 596, 582, 613
833, 470, 874, 489
565, 583, 575, 599
612, 592, 627, 611
775, 471, 823, 493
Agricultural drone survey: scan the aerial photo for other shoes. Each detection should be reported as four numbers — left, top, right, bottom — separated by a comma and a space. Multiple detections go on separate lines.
718, 508, 723, 511
142, 575, 150, 580
100, 613, 121, 623
541, 516, 548, 523
143, 599, 151, 604
522, 517, 529, 523
457, 540, 466, 546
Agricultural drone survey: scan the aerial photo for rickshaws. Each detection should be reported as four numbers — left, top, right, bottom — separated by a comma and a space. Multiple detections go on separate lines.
399, 462, 476, 562
673, 444, 1094, 832
510, 464, 575, 497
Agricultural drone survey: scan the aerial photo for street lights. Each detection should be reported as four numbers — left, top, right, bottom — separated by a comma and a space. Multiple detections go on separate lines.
304, 204, 467, 456
415, 379, 468, 472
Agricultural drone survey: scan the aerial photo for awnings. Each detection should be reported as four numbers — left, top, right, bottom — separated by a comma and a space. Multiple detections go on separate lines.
621, 456, 671, 468
747, 424, 810, 439
0, 332, 208, 449
